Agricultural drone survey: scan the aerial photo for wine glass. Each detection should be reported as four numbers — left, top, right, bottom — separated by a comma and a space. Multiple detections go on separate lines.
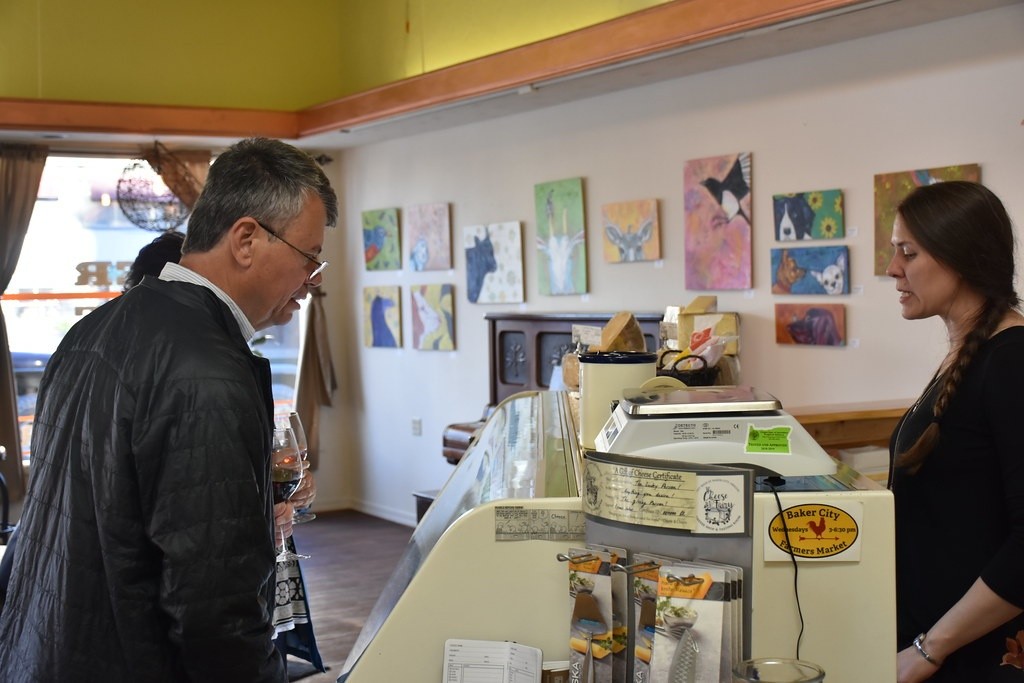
274, 411, 317, 524
272, 428, 311, 562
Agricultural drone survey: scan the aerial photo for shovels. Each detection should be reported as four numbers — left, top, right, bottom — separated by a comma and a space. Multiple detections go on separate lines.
572, 592, 608, 683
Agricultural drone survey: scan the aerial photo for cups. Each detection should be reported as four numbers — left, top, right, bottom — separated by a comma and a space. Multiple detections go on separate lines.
730, 657, 825, 683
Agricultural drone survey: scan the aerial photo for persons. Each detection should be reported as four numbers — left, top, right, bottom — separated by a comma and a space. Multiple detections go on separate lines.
122, 230, 327, 680
885, 180, 1024, 683
0, 136, 338, 683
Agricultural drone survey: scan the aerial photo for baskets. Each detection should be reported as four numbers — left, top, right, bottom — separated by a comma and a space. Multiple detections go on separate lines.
656, 350, 721, 387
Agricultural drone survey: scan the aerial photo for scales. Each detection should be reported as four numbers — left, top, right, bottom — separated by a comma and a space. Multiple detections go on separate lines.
593, 385, 836, 486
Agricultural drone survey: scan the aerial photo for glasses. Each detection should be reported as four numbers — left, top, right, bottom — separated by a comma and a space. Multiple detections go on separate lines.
255, 220, 329, 281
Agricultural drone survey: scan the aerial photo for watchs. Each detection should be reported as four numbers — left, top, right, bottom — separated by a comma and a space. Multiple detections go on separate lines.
913, 634, 939, 667
